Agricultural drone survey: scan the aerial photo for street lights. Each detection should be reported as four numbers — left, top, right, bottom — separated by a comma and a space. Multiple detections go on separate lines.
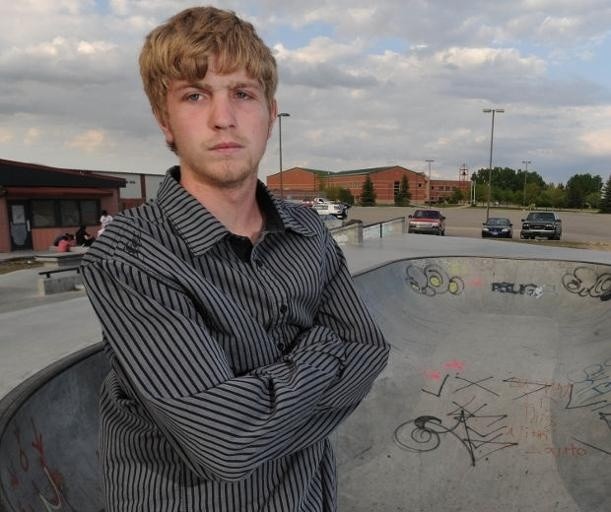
522, 160, 531, 206
277, 113, 290, 199
425, 160, 435, 209
482, 108, 505, 221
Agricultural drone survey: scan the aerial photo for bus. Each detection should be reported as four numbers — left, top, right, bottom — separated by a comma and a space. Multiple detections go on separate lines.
482, 218, 513, 238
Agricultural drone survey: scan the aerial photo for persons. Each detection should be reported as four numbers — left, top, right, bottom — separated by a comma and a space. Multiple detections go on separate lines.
97, 210, 114, 237
54, 233, 69, 246
58, 234, 74, 252
76, 225, 95, 247
79, 6, 391, 512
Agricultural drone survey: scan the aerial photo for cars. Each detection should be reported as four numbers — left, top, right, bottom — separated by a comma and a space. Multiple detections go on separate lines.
408, 209, 446, 236
281, 197, 352, 220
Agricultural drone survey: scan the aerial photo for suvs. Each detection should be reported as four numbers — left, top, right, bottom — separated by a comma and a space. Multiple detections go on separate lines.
520, 211, 562, 240
